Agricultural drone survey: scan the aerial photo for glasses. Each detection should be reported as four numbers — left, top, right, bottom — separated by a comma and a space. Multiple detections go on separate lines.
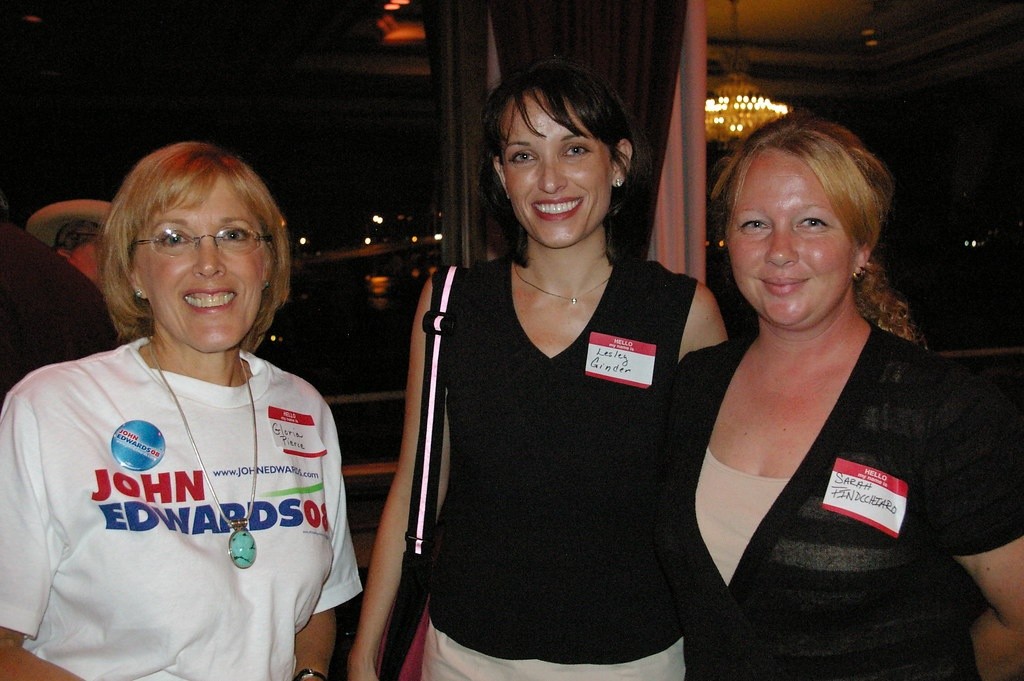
131, 228, 274, 255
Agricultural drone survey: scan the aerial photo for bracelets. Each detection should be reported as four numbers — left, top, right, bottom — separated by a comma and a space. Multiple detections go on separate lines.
294, 668, 327, 681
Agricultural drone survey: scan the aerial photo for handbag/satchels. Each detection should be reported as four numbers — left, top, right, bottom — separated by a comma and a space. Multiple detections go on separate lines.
376, 516, 430, 681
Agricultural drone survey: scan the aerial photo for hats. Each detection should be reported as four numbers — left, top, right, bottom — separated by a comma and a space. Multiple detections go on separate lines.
26, 200, 112, 248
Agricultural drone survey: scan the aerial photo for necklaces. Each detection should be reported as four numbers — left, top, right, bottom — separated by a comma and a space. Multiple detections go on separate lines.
512, 263, 609, 303
150, 343, 258, 569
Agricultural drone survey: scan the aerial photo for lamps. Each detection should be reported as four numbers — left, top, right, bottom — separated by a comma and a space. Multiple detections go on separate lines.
705, 1, 792, 147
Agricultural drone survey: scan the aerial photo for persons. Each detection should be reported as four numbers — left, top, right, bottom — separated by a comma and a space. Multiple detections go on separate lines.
0, 143, 363, 681
670, 112, 1024, 681
348, 59, 727, 681
